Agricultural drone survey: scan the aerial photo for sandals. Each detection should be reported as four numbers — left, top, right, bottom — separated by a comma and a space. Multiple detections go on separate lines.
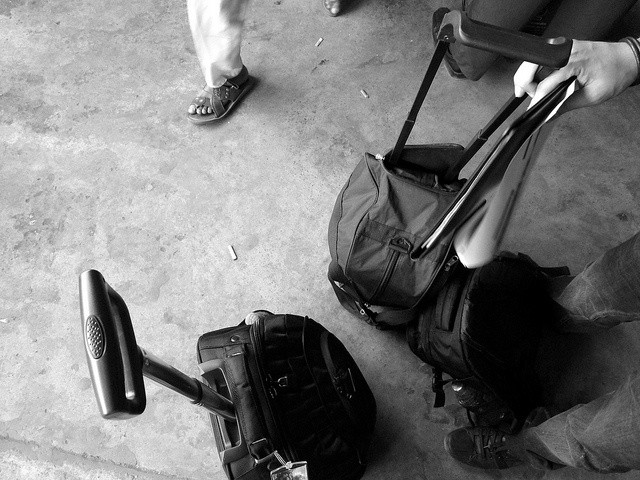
186, 77, 255, 125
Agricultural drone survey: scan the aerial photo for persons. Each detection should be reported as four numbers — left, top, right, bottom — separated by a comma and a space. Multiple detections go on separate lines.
442, 37, 640, 480
432, 0, 632, 82
186, 0, 343, 123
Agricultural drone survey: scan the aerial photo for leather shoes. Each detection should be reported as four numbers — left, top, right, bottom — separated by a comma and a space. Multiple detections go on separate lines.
432, 6, 466, 78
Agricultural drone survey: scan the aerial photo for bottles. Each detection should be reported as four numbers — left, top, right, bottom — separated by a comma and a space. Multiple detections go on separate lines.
450, 378, 488, 414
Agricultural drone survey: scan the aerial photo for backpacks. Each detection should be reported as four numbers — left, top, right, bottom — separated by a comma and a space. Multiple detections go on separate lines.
407, 249, 571, 431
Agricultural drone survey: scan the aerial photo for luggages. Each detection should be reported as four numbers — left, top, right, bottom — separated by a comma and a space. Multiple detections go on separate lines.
325, 9, 573, 332
79, 269, 375, 480
